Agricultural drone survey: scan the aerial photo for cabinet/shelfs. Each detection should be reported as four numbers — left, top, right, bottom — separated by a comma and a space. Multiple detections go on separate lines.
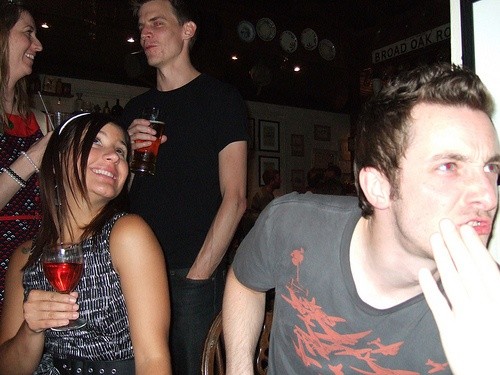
38, 90, 74, 117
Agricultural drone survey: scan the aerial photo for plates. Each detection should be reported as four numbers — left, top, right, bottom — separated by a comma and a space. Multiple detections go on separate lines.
317, 39, 336, 60
280, 30, 298, 53
301, 28, 318, 52
256, 17, 276, 40
236, 20, 256, 42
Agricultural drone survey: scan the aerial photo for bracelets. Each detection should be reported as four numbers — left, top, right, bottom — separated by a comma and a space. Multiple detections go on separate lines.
0, 166, 27, 188
20, 151, 40, 174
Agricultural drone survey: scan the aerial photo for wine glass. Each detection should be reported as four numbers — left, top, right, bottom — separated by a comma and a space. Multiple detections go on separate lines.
42, 245, 86, 331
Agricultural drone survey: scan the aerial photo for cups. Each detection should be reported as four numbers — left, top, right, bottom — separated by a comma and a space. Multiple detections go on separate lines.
129, 106, 167, 177
372, 78, 382, 97
48, 113, 73, 132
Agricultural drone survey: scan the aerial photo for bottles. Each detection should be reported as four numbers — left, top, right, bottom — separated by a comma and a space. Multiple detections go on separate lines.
73, 92, 123, 115
32, 74, 69, 95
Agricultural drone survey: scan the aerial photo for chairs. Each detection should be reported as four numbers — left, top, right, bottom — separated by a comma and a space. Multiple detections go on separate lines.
199, 288, 274, 375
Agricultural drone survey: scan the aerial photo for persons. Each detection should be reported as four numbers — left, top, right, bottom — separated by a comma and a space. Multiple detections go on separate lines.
223, 60, 500, 375
0, 0, 58, 327
116, 0, 255, 375
0, 112, 172, 375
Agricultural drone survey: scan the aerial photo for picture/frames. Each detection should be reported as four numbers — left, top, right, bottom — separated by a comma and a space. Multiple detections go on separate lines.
290, 134, 305, 157
259, 118, 281, 153
259, 156, 281, 189
314, 124, 331, 142
310, 148, 337, 168
250, 118, 256, 150
292, 168, 305, 192
